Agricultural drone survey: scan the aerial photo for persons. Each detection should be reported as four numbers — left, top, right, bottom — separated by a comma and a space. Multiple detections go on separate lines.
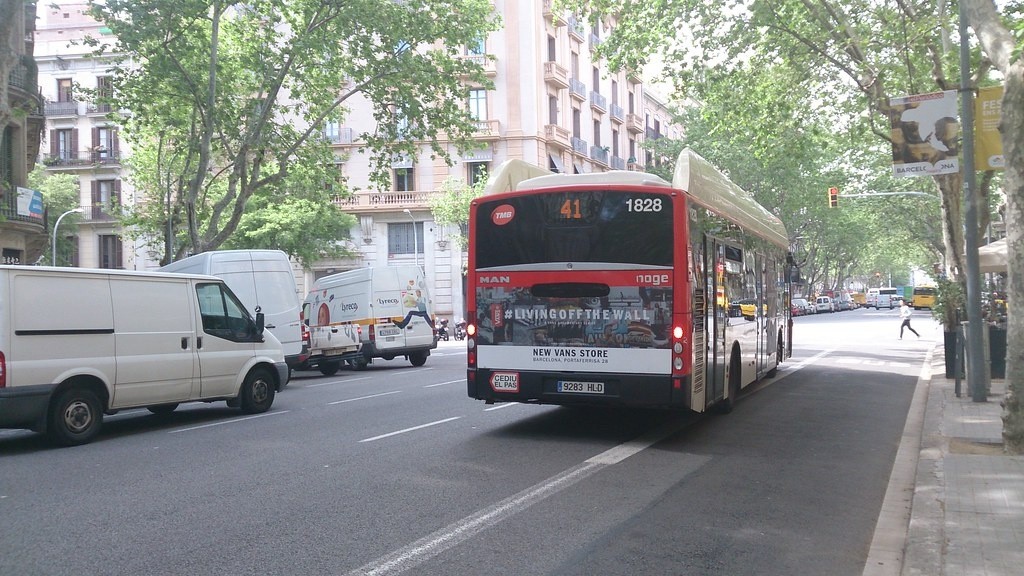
476, 313, 494, 338
595, 324, 621, 348
654, 303, 667, 340
898, 299, 920, 340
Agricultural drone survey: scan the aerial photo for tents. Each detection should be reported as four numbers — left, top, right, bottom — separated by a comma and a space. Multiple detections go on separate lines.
960, 237, 1009, 304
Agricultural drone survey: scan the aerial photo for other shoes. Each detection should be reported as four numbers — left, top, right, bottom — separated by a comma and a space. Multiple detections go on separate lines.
898, 338, 901, 340
918, 334, 920, 338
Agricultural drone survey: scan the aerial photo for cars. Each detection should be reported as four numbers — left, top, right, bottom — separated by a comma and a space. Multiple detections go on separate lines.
788, 286, 912, 318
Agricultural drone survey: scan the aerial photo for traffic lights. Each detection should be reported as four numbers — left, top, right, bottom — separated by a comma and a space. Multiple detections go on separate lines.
829, 187, 838, 209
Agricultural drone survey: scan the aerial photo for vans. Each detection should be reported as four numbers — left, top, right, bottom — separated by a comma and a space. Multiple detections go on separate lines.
0, 265, 289, 447
154, 249, 302, 357
292, 266, 437, 371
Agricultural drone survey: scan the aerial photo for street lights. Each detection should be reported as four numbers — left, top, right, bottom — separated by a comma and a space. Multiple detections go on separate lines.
404, 209, 418, 266
52, 208, 84, 267
166, 133, 227, 268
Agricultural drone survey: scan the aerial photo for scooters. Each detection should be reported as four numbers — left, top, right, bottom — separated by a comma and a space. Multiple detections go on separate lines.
435, 317, 449, 341
453, 316, 466, 342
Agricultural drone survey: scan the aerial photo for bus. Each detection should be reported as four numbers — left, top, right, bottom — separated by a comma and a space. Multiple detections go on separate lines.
466, 147, 800, 417
911, 286, 937, 311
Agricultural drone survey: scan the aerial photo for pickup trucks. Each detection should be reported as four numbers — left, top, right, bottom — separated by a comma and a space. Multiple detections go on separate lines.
295, 323, 363, 376
